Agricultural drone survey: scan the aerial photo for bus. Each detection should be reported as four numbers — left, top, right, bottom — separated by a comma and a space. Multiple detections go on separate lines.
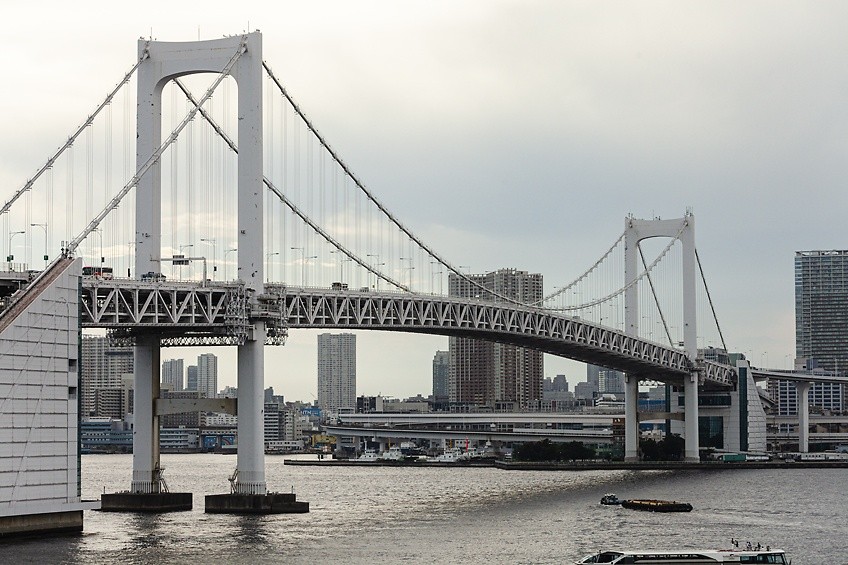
141, 271, 165, 283
82, 266, 112, 280
331, 282, 349, 291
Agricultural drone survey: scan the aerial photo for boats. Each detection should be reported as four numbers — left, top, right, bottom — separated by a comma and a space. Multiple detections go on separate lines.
621, 499, 693, 512
600, 493, 621, 505
576, 538, 792, 565
358, 442, 485, 462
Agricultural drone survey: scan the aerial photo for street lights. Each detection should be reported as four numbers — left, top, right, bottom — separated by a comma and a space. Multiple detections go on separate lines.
484, 271, 602, 324
7, 224, 48, 272
91, 227, 103, 278
609, 305, 679, 349
697, 337, 754, 371
291, 247, 471, 300
760, 352, 793, 374
179, 239, 237, 280
266, 252, 280, 285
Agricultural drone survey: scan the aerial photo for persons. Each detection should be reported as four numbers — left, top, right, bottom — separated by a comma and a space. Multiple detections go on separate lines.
734, 540, 738, 549
747, 542, 751, 548
754, 542, 761, 551
731, 538, 734, 549
